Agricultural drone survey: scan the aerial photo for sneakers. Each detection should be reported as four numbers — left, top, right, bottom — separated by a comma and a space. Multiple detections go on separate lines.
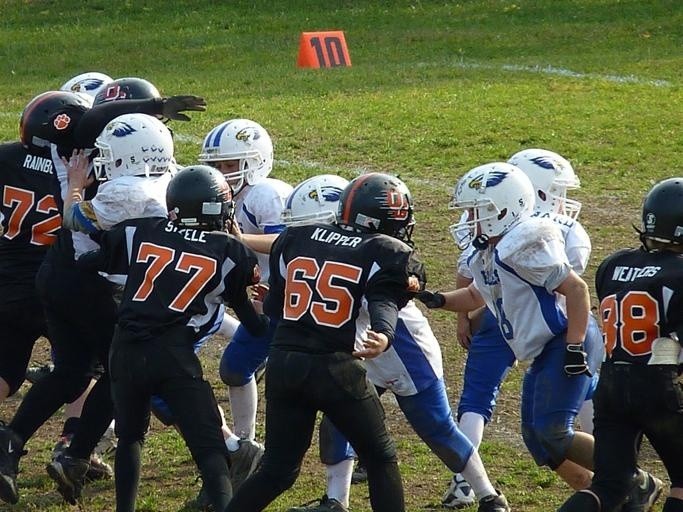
50, 442, 114, 480
230, 436, 265, 495
287, 493, 350, 512
46, 449, 90, 506
26, 363, 54, 385
0, 422, 28, 506
626, 467, 664, 512
195, 476, 233, 512
477, 489, 512, 512
440, 474, 476, 506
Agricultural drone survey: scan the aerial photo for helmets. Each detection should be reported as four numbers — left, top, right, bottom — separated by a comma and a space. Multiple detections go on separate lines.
58, 71, 114, 97
92, 113, 174, 184
447, 161, 535, 251
278, 174, 350, 228
197, 118, 274, 198
18, 90, 90, 156
507, 148, 582, 228
336, 171, 416, 242
165, 164, 237, 231
635, 177, 683, 250
90, 76, 160, 106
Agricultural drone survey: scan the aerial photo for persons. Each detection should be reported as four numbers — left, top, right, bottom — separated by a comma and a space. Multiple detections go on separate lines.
23, 68, 114, 389
0, 76, 208, 505
0, 92, 114, 485
224, 172, 411, 512
439, 147, 602, 511
277, 175, 510, 512
409, 162, 664, 511
59, 113, 270, 500
106, 166, 270, 512
200, 120, 296, 447
556, 177, 683, 512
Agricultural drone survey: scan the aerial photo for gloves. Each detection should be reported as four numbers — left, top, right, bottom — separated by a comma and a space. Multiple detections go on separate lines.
159, 93, 208, 122
563, 341, 593, 381
415, 287, 447, 310
